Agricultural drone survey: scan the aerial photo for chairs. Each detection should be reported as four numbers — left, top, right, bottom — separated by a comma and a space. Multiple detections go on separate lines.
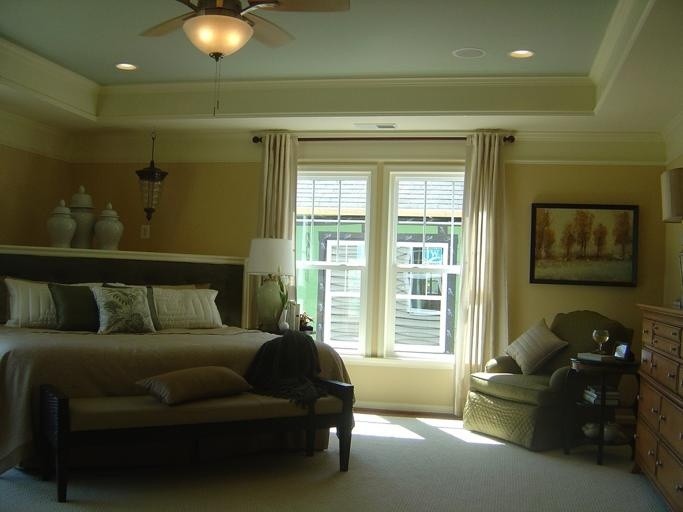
462, 311, 635, 453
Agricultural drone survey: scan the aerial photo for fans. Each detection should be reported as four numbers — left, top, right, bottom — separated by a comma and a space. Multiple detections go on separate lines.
137, 0, 352, 51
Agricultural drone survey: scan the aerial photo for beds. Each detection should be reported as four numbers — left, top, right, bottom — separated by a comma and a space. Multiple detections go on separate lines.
1, 243, 355, 478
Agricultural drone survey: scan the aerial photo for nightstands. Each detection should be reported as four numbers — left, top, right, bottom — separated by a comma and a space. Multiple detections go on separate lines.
250, 325, 317, 337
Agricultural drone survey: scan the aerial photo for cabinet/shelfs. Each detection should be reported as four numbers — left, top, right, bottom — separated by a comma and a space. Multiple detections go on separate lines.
563, 357, 640, 465
631, 300, 682, 511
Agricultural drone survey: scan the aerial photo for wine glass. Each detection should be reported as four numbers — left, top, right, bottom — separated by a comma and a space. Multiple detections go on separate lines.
593, 328, 610, 355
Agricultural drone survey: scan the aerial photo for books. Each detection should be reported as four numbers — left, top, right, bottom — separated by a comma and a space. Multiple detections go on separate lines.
582, 384, 623, 406
614, 408, 635, 425
577, 354, 614, 362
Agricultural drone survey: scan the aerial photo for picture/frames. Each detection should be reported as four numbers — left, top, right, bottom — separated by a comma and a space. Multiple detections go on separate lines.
612, 340, 630, 361
528, 201, 643, 287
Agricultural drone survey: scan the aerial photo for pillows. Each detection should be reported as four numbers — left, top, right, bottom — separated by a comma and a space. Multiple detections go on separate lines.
503, 316, 569, 375
3, 275, 223, 335
136, 366, 251, 405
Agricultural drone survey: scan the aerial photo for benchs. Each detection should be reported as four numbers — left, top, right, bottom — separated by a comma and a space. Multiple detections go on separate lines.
31, 378, 356, 503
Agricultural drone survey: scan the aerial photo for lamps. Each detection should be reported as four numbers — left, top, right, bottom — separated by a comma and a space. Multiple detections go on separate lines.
661, 168, 682, 224
181, 0, 255, 60
247, 238, 296, 331
135, 134, 170, 220
